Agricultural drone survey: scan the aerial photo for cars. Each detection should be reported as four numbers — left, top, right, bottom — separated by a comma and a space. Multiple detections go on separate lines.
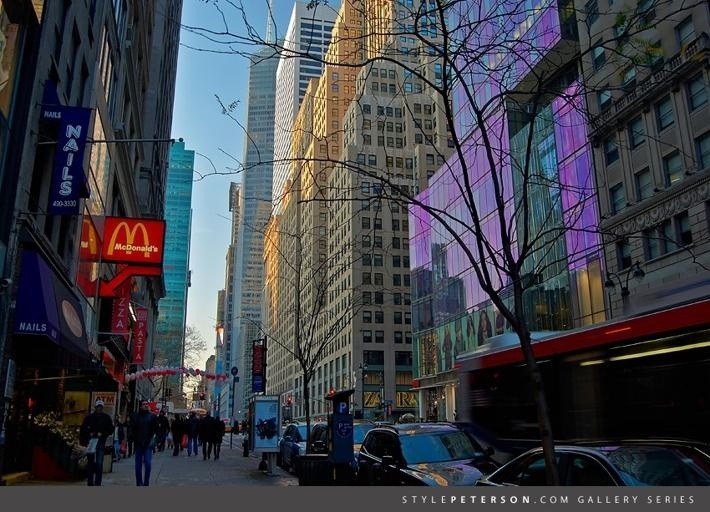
222, 417, 250, 434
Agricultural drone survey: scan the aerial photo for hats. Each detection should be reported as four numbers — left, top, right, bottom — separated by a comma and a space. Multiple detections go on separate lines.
96, 400, 104, 407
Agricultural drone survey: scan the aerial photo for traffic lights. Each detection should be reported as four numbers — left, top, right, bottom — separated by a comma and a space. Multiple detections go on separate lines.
200, 392, 204, 400
166, 388, 169, 396
288, 395, 292, 404
168, 388, 172, 396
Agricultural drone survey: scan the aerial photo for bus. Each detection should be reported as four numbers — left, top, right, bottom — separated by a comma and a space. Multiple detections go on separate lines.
452, 285, 709, 463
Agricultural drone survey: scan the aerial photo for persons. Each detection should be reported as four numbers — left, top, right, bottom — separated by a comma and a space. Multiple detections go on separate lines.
128, 398, 163, 485
79, 400, 114, 486
113, 410, 225, 463
442, 307, 505, 371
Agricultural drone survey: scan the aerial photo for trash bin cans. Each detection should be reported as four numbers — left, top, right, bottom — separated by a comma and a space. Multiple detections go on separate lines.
296, 453, 334, 486
102, 446, 114, 473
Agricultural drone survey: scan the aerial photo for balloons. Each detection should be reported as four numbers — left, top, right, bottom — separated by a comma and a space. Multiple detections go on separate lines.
148, 401, 161, 415
119, 365, 227, 384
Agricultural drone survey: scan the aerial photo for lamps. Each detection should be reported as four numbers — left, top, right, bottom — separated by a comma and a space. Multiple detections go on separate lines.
603, 260, 644, 299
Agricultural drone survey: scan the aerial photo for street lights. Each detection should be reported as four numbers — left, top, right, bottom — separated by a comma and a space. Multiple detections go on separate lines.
358, 361, 369, 420
604, 260, 646, 319
